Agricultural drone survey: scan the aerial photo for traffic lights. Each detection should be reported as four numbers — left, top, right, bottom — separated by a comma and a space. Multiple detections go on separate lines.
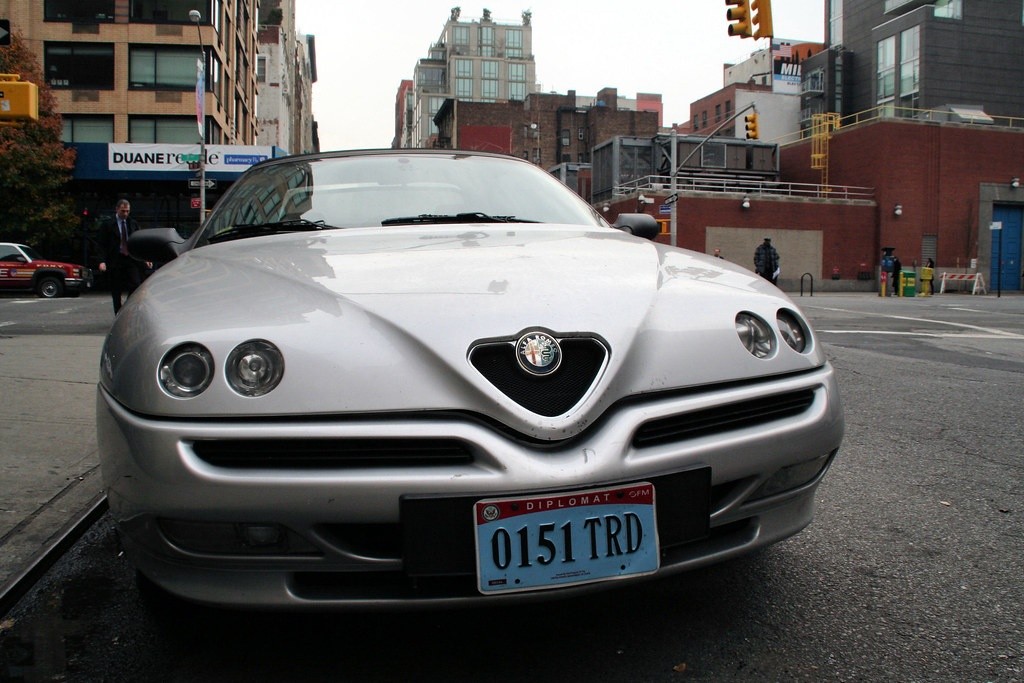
745, 111, 759, 140
751, 0, 774, 40
726, 0, 753, 39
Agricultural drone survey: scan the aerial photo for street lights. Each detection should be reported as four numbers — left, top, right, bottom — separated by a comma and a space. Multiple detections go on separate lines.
189, 10, 206, 226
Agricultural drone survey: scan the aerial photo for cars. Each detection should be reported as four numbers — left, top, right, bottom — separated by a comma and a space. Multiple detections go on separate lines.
98, 146, 845, 607
0, 242, 89, 298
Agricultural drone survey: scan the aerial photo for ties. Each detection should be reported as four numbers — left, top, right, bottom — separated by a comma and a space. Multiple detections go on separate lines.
120, 220, 128, 257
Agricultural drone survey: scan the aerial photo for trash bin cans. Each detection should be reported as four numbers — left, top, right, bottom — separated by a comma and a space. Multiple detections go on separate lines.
898, 269, 916, 297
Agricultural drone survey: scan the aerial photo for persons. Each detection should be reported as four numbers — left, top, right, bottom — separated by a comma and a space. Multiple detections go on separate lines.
891, 257, 902, 295
95, 199, 152, 316
713, 248, 724, 259
754, 237, 780, 286
926, 258, 934, 295
